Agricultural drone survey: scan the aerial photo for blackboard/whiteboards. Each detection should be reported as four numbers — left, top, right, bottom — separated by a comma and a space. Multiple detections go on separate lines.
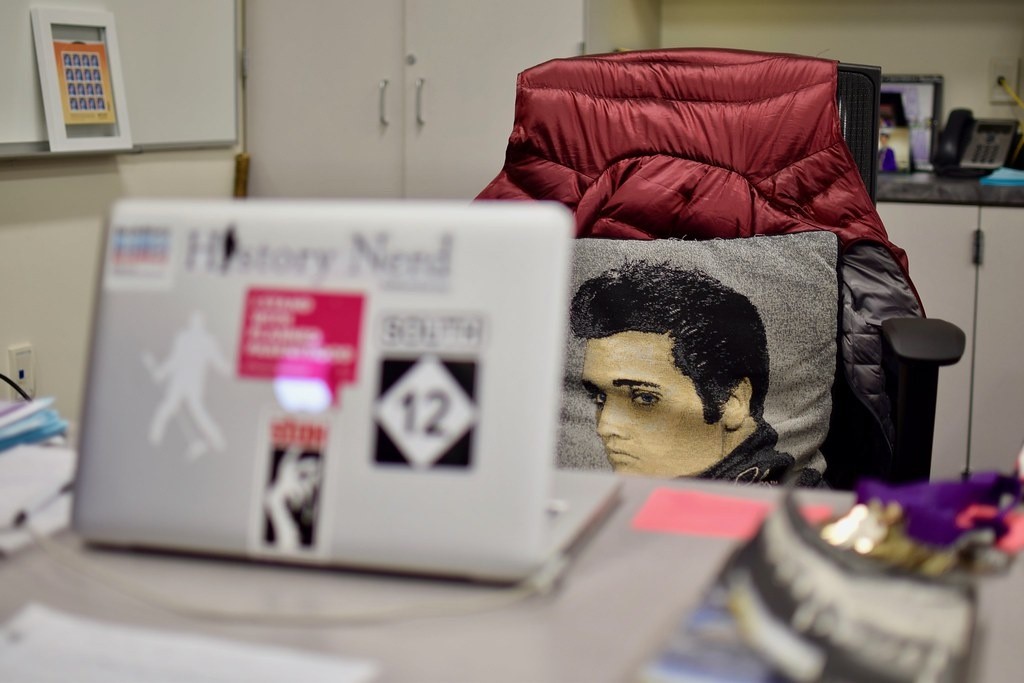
0, 0, 248, 160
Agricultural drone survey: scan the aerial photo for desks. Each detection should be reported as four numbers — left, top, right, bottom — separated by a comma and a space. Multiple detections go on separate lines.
0, 464, 1023, 683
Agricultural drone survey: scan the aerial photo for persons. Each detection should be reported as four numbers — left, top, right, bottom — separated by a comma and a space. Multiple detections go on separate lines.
878, 134, 897, 171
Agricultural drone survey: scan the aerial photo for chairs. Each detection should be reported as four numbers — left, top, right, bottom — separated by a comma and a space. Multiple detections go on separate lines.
510, 49, 968, 491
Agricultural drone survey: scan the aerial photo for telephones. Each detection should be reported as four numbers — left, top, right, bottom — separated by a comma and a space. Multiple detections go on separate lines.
931, 108, 1020, 176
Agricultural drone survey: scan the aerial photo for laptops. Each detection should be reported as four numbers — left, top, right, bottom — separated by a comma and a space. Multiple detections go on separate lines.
76, 195, 627, 584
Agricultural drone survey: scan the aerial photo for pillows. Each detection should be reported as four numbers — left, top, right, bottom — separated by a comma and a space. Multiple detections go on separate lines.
551, 231, 843, 494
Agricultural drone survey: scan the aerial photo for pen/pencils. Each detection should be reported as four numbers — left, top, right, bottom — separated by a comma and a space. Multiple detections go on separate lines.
996, 74, 1024, 107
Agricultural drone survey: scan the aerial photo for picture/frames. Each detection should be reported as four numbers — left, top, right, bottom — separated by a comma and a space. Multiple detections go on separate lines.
29, 4, 133, 154
876, 71, 945, 170
